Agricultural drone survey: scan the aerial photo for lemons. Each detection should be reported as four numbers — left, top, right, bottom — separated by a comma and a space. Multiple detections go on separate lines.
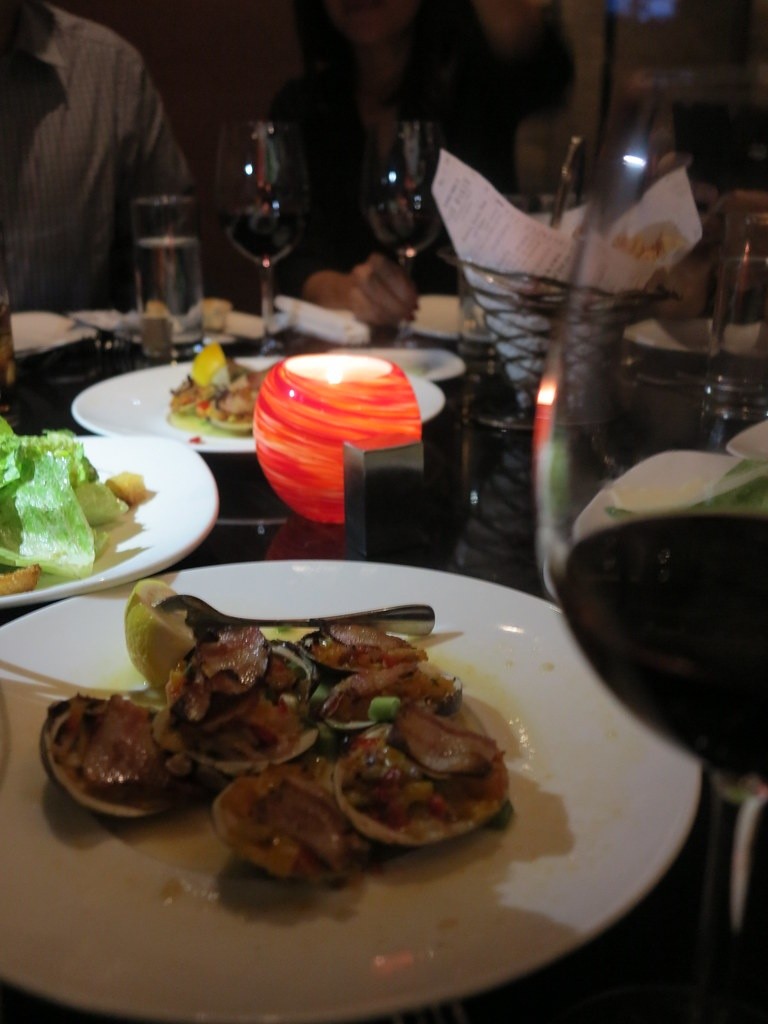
191, 340, 230, 386
125, 578, 194, 690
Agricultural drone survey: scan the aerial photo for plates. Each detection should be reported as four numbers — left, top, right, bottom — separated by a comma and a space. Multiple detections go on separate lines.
0, 434, 220, 608
627, 316, 753, 351
725, 419, 768, 462
0, 561, 702, 1023
571, 451, 744, 541
70, 357, 446, 453
330, 346, 466, 381
414, 291, 488, 341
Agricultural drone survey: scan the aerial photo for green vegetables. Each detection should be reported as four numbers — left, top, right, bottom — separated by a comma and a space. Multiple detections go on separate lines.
0, 413, 130, 581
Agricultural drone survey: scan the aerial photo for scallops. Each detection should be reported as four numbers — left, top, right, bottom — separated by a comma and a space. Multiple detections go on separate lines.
40, 626, 510, 882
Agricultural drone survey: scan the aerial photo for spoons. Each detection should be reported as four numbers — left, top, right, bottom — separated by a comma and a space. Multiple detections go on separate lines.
156, 596, 436, 645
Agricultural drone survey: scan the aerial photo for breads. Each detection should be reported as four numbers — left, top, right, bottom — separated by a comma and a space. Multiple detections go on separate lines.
146, 296, 233, 329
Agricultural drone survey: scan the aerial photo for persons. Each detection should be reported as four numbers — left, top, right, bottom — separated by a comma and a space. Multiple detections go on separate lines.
220, 0, 576, 329
0, 0, 199, 311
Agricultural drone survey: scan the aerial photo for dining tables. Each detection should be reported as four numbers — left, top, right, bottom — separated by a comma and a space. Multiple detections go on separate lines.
0, 329, 768, 1024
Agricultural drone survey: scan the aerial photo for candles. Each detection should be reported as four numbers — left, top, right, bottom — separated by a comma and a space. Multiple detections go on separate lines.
251, 352, 422, 525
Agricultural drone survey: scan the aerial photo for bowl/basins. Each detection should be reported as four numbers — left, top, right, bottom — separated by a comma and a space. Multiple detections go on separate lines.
612, 324, 710, 447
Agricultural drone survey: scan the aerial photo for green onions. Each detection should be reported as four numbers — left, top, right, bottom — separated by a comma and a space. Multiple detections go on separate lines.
275, 622, 401, 751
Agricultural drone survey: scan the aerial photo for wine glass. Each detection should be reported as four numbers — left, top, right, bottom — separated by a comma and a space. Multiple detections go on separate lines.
361, 122, 444, 348
214, 117, 310, 356
533, 1, 768, 1024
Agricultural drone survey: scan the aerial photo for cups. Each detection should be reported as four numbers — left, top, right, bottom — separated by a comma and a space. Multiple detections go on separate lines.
698, 210, 768, 420
132, 196, 203, 357
0, 277, 21, 427
255, 353, 423, 524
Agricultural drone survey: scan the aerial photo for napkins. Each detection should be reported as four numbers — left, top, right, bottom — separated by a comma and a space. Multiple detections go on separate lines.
273, 294, 371, 348
